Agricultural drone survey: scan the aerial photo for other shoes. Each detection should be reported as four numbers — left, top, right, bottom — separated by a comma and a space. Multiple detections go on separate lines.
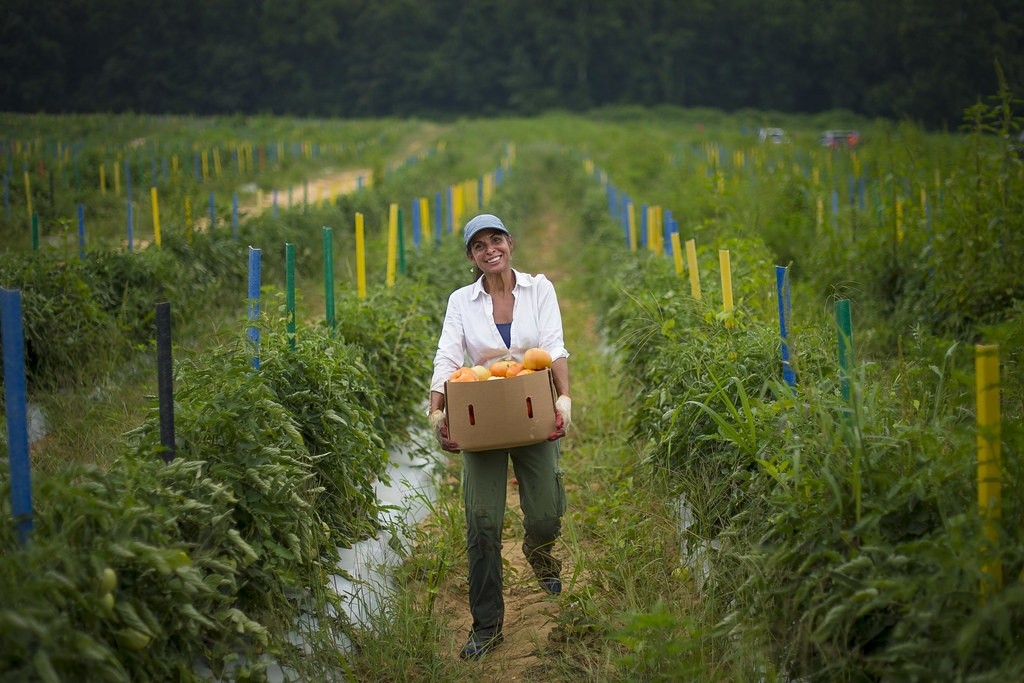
461, 632, 504, 660
522, 538, 561, 596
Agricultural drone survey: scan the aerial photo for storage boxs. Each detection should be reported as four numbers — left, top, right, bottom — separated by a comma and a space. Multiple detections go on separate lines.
443, 367, 559, 452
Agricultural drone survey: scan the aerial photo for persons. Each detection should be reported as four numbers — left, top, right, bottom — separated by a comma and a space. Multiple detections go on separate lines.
429, 212, 572, 661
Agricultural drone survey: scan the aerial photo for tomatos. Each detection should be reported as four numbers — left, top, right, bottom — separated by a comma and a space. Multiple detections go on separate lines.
670, 565, 690, 579
448, 346, 553, 424
99, 567, 150, 650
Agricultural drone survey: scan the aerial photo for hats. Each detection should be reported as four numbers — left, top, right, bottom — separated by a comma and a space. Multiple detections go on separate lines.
464, 214, 509, 247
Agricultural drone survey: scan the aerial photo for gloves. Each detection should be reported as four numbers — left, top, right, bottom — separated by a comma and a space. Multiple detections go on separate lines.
548, 395, 572, 441
430, 410, 461, 454
